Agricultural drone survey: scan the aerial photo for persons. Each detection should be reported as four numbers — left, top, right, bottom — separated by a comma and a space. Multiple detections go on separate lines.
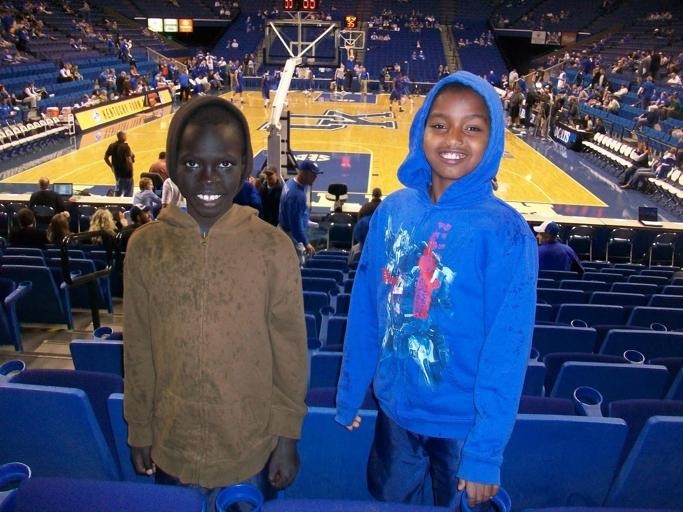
334, 70, 539, 511
0, 0, 683, 282
122, 95, 312, 512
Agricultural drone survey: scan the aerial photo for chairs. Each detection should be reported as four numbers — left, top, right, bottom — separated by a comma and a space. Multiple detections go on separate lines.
0, 0, 195, 161
0, 199, 682, 511
198, 1, 511, 92
515, 1, 680, 215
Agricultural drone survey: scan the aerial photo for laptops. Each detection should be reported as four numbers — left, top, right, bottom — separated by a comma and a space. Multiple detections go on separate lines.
640, 205, 663, 227
53, 183, 73, 200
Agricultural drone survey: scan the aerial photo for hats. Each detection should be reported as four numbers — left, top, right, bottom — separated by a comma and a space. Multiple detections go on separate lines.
533, 221, 558, 234
130, 204, 150, 219
299, 160, 323, 174
